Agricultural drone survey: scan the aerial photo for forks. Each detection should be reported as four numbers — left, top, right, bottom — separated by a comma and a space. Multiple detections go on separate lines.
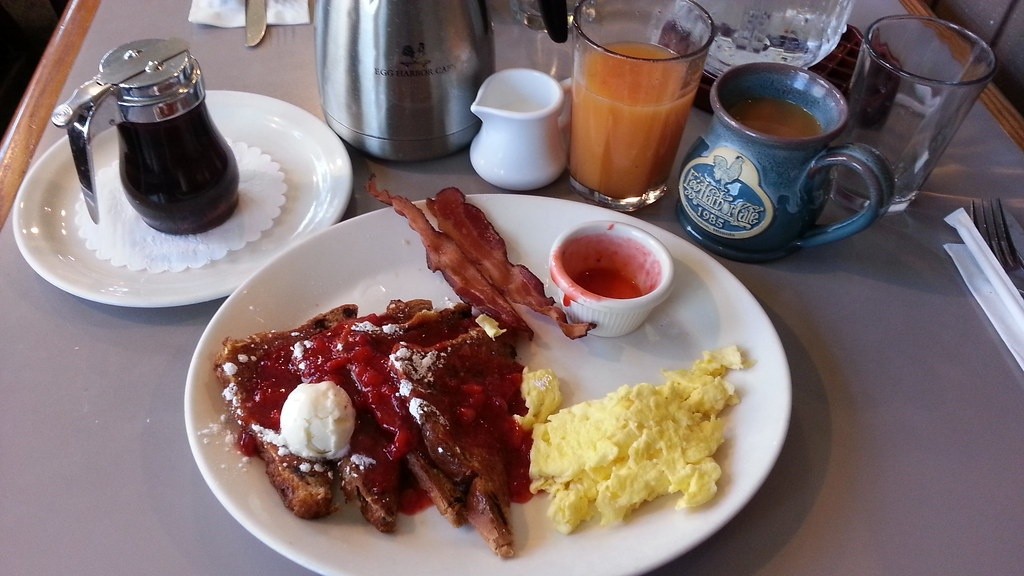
968, 193, 1024, 312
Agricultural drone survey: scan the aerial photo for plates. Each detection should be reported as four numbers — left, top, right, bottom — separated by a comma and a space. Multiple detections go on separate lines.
8, 91, 356, 312
180, 194, 794, 574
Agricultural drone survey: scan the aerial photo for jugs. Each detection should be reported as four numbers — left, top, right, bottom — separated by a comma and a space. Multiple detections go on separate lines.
306, 0, 569, 161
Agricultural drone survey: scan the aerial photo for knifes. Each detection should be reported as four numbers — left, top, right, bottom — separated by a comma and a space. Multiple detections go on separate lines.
241, 0, 268, 49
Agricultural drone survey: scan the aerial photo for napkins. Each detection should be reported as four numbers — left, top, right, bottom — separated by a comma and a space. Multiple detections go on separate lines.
186, 0, 311, 29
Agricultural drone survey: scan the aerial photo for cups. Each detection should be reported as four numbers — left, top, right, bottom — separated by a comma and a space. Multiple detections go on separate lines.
55, 35, 238, 238
468, 2, 997, 336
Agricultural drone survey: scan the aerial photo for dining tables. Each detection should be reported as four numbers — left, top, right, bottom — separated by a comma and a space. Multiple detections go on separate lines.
0, 0, 1024, 576
